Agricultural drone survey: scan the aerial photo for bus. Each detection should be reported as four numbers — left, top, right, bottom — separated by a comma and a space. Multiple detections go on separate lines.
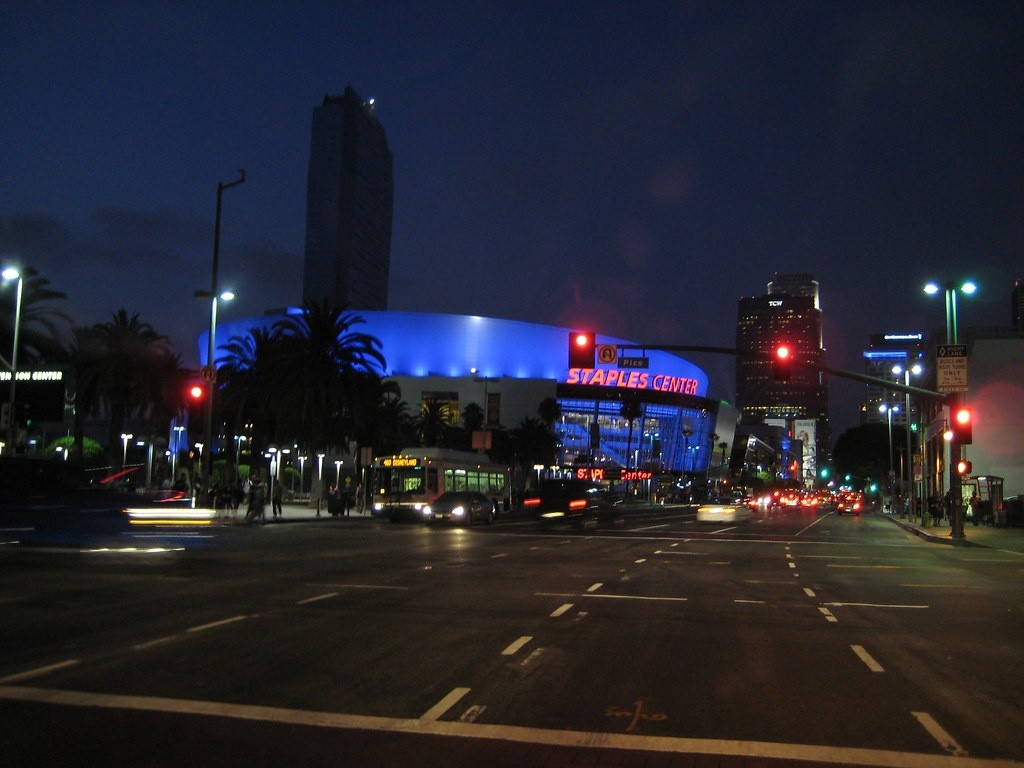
370, 447, 513, 522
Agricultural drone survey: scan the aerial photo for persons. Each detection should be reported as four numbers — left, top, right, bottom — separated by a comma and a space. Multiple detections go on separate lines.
272, 480, 283, 518
242, 472, 267, 522
969, 491, 980, 526
355, 481, 364, 514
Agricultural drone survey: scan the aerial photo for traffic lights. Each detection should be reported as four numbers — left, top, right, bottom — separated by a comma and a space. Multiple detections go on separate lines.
191, 387, 201, 398
957, 461, 972, 476
954, 408, 972, 445
568, 331, 595, 370
773, 344, 791, 381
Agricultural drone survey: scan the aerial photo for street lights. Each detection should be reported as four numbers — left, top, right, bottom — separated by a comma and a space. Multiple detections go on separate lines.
2, 266, 24, 453
137, 436, 344, 508
473, 376, 499, 452
923, 282, 976, 538
921, 417, 953, 527
892, 365, 921, 523
879, 405, 899, 514
195, 290, 235, 509
121, 433, 133, 472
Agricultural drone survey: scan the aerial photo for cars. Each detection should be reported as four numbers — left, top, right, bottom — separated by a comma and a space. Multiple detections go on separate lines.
423, 491, 496, 524
1002, 496, 1024, 527
758, 489, 862, 516
520, 478, 610, 526
0, 453, 190, 542
697, 496, 751, 524
